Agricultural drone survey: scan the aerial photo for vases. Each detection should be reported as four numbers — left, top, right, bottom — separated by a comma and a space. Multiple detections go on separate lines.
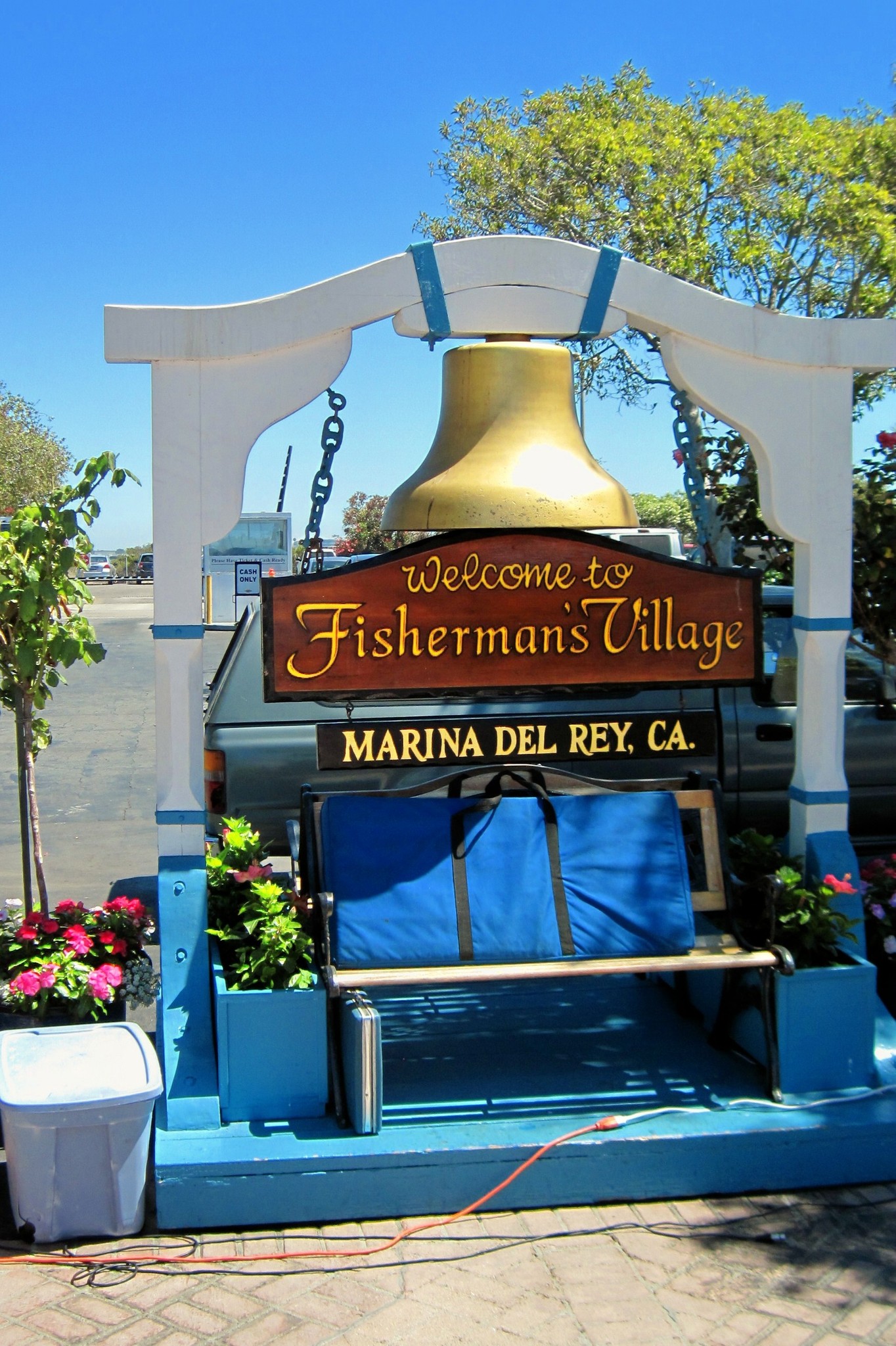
713, 947, 877, 1092
207, 943, 328, 1123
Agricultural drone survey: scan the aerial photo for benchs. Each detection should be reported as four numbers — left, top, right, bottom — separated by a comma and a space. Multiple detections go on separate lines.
293, 763, 793, 1130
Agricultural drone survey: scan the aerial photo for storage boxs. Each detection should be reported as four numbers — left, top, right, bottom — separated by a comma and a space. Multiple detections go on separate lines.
0, 1024, 165, 1245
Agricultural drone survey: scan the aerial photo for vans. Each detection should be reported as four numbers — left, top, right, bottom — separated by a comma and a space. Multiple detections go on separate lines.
199, 578, 896, 858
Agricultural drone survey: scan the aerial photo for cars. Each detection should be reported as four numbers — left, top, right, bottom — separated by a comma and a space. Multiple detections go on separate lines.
295, 549, 336, 576
591, 526, 692, 562
341, 554, 380, 565
691, 535, 786, 575
133, 553, 154, 584
77, 555, 118, 585
300, 556, 349, 574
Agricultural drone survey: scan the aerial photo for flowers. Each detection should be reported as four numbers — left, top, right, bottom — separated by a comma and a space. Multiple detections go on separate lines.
204, 815, 315, 984
0, 893, 164, 1022
734, 828, 856, 959
